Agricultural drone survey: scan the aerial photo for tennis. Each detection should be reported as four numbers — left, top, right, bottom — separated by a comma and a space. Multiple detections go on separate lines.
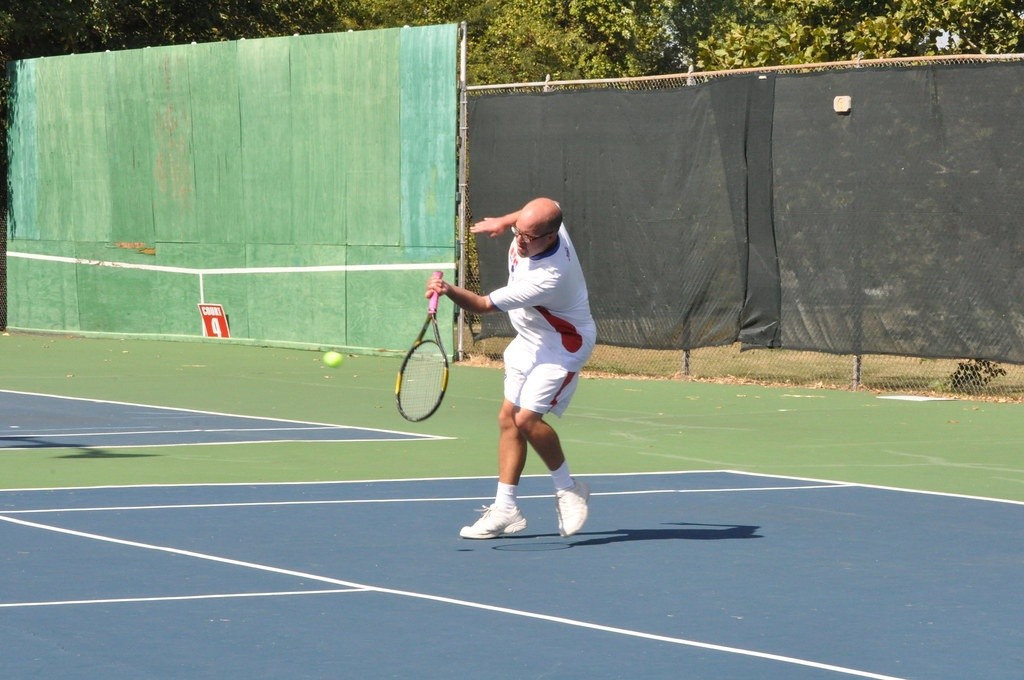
321, 351, 344, 369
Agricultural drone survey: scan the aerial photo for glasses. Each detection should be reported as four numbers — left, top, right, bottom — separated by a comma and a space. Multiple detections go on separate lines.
511, 224, 552, 244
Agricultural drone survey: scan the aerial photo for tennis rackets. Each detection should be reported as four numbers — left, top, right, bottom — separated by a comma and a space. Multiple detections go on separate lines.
394, 270, 451, 423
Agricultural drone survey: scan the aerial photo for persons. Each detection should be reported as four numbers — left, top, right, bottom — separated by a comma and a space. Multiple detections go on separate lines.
425, 197, 597, 539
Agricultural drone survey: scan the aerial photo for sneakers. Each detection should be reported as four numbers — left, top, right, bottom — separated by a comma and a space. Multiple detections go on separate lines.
554, 477, 590, 537
460, 504, 527, 540
